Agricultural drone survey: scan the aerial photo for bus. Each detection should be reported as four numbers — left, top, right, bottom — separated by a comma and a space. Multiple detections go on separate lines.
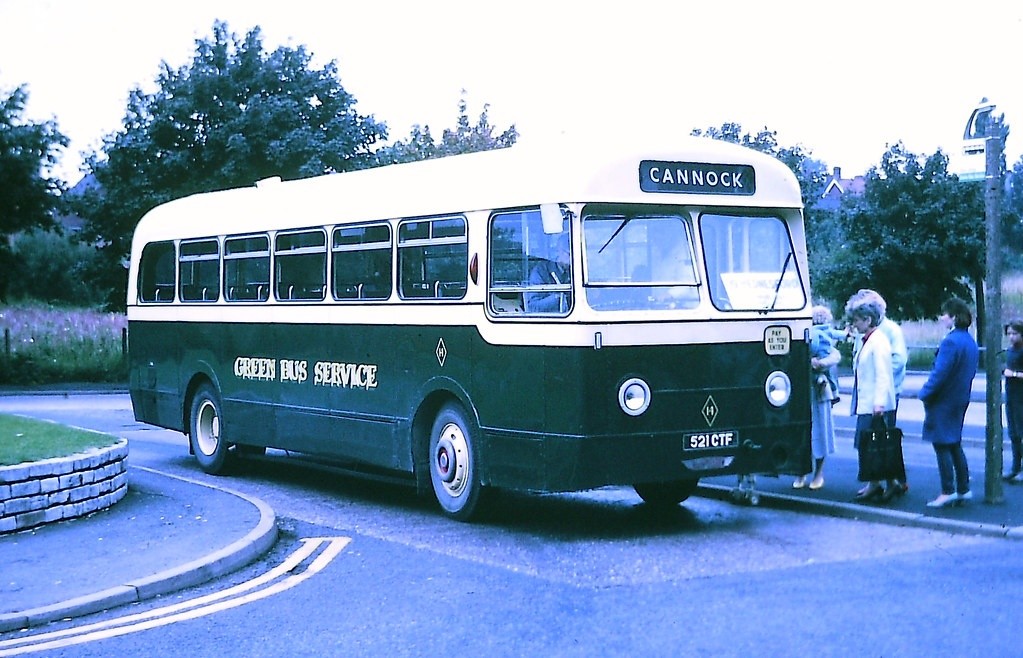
119, 129, 814, 526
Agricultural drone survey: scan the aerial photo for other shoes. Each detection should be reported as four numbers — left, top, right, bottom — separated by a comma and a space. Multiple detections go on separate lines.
1005, 468, 1023, 481
809, 477, 824, 489
792, 474, 806, 489
855, 481, 907, 504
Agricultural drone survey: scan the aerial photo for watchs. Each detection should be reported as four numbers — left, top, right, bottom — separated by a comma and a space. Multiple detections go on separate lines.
1012, 371, 1017, 378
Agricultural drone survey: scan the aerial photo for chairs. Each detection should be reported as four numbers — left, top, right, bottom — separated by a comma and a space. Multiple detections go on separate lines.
152, 278, 523, 316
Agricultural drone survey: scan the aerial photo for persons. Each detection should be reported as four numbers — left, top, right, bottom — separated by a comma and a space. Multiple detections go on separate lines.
916, 297, 980, 508
846, 289, 910, 495
851, 299, 898, 503
527, 231, 574, 314
811, 306, 848, 395
792, 340, 840, 490
1003, 319, 1023, 482
632, 265, 653, 297
649, 224, 703, 298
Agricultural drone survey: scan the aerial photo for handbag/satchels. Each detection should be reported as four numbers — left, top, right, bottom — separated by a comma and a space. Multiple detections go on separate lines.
858, 414, 904, 482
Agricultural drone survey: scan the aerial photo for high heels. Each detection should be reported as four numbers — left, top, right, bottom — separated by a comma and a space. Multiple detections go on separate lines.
927, 491, 957, 509
951, 491, 973, 505
